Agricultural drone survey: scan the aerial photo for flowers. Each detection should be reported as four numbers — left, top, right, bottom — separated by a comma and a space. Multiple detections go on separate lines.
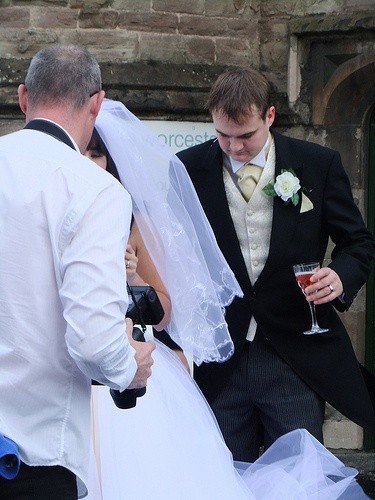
262, 168, 301, 207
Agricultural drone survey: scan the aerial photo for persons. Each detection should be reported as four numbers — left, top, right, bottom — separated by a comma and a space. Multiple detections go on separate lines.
82, 118, 174, 499
0, 42, 156, 500
168, 66, 375, 472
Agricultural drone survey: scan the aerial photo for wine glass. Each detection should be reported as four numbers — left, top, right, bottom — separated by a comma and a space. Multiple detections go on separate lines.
294, 262, 329, 335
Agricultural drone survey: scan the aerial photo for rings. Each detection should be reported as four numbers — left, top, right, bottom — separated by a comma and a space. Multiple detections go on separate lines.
126, 261, 130, 268
328, 284, 333, 292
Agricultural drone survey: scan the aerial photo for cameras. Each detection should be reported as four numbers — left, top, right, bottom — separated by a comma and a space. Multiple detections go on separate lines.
90, 286, 165, 409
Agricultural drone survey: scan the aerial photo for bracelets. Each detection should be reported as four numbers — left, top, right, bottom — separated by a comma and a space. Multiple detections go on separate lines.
145, 283, 150, 287
